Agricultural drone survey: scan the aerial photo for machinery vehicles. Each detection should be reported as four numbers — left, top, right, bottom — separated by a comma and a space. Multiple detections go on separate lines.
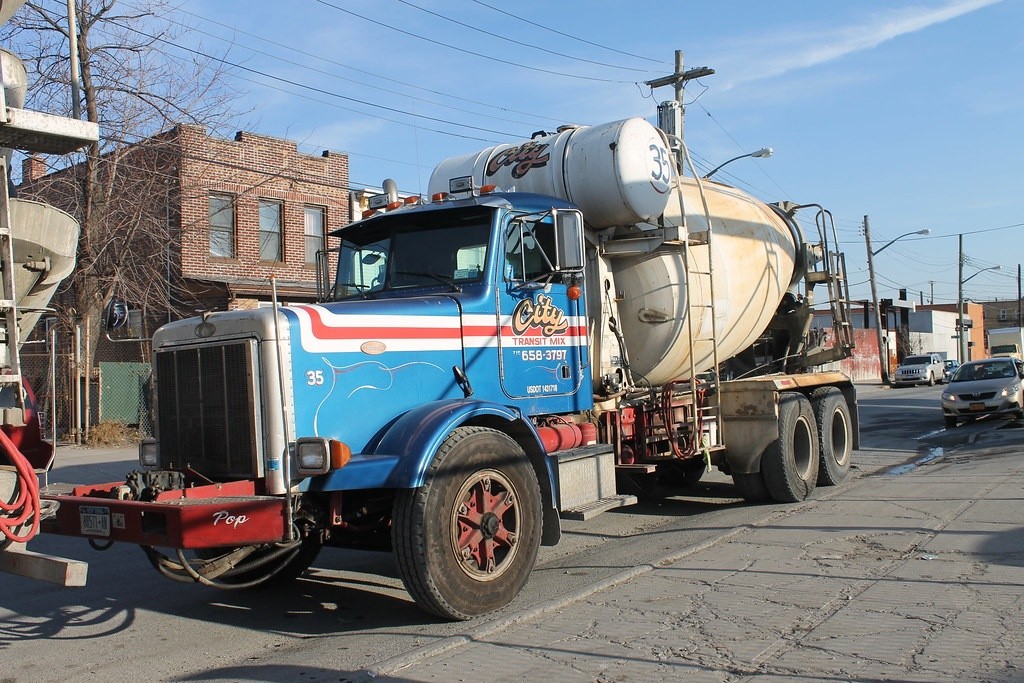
33, 116, 859, 621
0, 0, 97, 588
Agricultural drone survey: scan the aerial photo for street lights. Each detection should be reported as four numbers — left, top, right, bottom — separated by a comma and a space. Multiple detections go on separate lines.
865, 229, 930, 384
703, 149, 775, 183
959, 265, 1002, 365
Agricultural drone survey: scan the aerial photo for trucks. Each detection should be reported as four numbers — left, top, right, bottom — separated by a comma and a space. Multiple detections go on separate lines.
987, 327, 1024, 359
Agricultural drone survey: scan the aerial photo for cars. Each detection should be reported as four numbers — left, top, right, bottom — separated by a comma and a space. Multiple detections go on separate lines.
941, 356, 1024, 425
895, 354, 945, 386
942, 359, 960, 378
